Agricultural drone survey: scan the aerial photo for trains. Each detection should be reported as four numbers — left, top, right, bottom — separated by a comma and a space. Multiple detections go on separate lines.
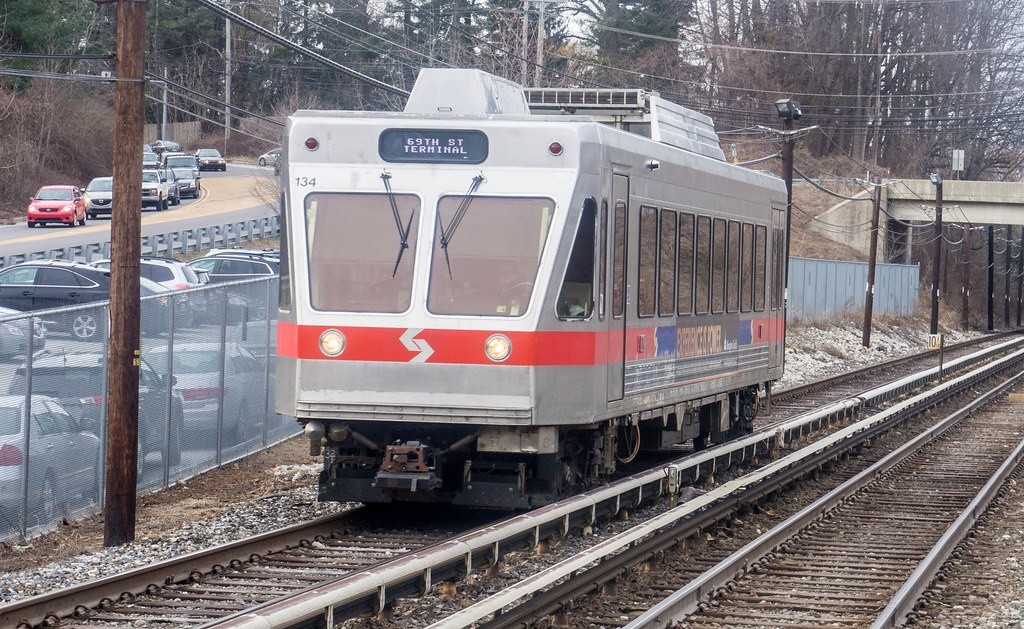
276, 67, 785, 510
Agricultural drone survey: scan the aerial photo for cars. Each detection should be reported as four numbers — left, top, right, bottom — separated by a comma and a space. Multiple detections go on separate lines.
141, 342, 271, 442
258, 148, 283, 167
26, 186, 86, 228
195, 149, 225, 172
80, 177, 112, 220
232, 322, 277, 373
0, 395, 100, 529
0, 245, 291, 360
141, 140, 201, 211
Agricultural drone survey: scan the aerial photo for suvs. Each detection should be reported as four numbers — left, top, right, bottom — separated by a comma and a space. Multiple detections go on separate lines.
8, 343, 184, 483
